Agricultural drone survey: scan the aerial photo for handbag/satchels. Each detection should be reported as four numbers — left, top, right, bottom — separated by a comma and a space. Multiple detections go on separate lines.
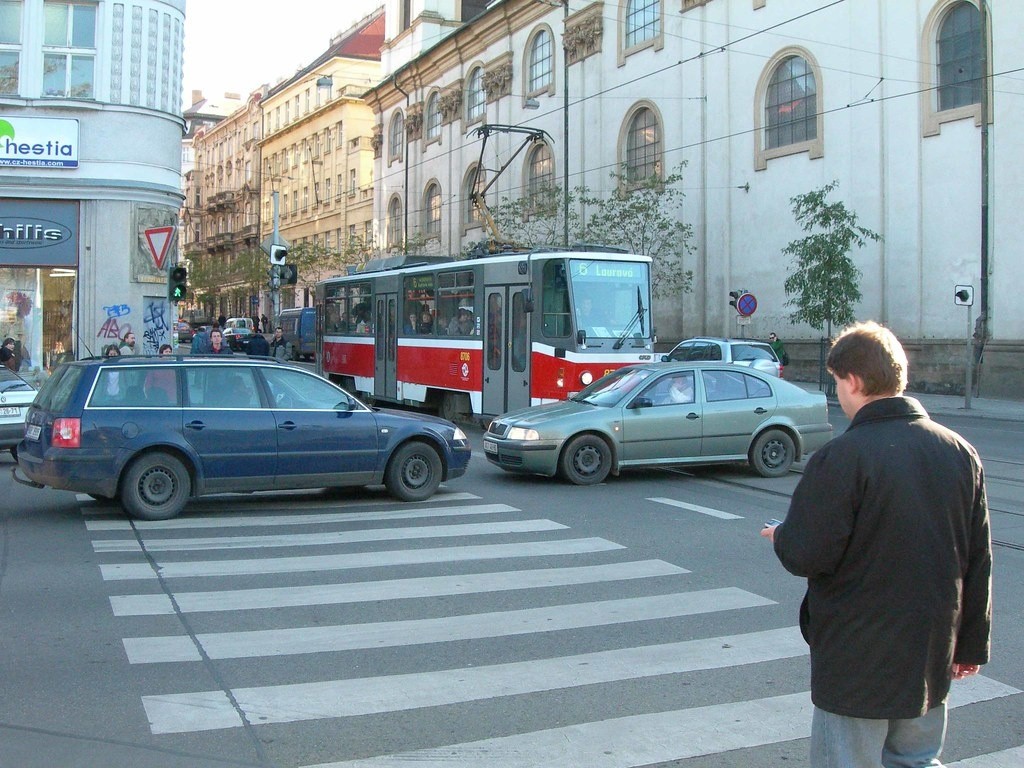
783, 351, 788, 365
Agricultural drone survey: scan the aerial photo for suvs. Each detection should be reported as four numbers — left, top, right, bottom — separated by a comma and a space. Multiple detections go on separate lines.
11, 355, 473, 521
660, 335, 784, 380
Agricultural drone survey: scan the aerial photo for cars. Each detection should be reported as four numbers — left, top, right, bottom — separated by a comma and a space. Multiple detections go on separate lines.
482, 362, 833, 485
0, 364, 38, 462
192, 326, 211, 339
178, 319, 194, 343
222, 328, 253, 352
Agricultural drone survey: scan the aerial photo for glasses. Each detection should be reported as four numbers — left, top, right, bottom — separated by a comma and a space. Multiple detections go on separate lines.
770, 337, 774, 339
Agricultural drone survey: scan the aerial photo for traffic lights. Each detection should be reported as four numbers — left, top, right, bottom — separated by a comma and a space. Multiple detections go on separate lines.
169, 266, 187, 301
729, 289, 744, 309
955, 285, 974, 305
271, 244, 287, 266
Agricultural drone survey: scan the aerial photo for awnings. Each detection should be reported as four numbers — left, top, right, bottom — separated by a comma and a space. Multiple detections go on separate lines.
354, 251, 454, 276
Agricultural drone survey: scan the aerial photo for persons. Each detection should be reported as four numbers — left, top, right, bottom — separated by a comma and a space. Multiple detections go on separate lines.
190, 326, 210, 357
489, 293, 528, 369
2, 291, 31, 363
200, 322, 233, 358
217, 312, 227, 328
233, 375, 261, 407
437, 316, 450, 336
403, 312, 420, 335
758, 319, 993, 768
445, 306, 473, 335
660, 376, 695, 404
419, 311, 435, 335
767, 331, 785, 367
575, 296, 597, 330
118, 331, 146, 402
245, 332, 270, 361
261, 313, 268, 333
267, 326, 293, 363
333, 312, 357, 333
252, 313, 260, 333
0, 337, 15, 371
102, 342, 127, 402
146, 344, 176, 403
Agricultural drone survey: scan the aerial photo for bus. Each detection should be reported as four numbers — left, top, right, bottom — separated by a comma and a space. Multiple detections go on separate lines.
278, 307, 318, 361
312, 124, 657, 429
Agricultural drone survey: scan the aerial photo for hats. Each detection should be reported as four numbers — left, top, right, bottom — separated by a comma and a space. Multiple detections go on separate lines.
255, 329, 262, 334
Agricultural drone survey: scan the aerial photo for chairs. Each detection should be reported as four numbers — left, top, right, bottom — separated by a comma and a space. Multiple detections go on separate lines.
120, 386, 147, 406
148, 387, 170, 406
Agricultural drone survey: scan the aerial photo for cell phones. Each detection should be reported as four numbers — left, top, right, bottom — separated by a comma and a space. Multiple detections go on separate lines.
765, 518, 784, 528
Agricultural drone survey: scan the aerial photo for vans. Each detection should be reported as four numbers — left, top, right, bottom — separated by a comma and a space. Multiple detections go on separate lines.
224, 318, 254, 332
